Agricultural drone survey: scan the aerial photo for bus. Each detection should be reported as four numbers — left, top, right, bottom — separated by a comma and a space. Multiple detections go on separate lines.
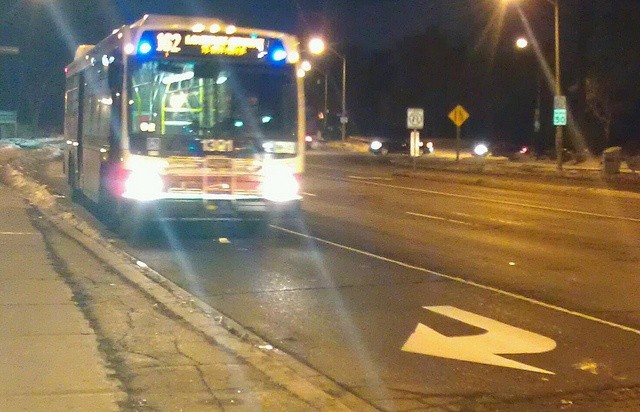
64, 13, 306, 239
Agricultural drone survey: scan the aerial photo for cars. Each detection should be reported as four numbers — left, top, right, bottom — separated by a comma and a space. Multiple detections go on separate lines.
365, 132, 436, 158
469, 139, 528, 161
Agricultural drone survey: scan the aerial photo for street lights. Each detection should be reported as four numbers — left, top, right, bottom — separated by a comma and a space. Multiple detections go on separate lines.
298, 70, 322, 139
514, 36, 542, 162
310, 38, 348, 144
301, 63, 328, 141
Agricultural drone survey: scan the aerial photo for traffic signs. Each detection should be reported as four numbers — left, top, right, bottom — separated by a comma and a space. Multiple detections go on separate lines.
448, 105, 470, 127
553, 109, 567, 126
407, 108, 424, 128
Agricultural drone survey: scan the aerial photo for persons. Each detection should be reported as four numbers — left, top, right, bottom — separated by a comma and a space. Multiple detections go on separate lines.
240, 90, 265, 140
